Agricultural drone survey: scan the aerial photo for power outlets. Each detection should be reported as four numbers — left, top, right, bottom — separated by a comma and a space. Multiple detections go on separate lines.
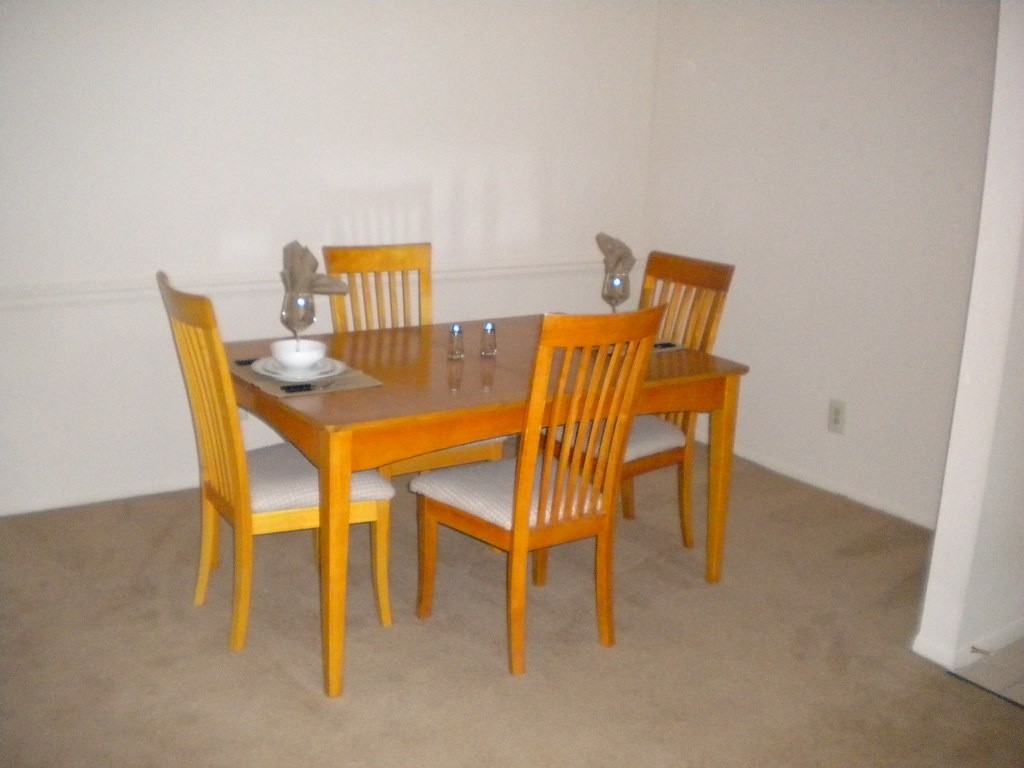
825, 399, 847, 434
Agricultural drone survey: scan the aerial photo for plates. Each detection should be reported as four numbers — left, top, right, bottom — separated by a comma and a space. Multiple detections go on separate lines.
250, 358, 346, 380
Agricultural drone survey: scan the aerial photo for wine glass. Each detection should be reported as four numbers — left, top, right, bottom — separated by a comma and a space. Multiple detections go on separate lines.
281, 291, 315, 351
602, 273, 630, 314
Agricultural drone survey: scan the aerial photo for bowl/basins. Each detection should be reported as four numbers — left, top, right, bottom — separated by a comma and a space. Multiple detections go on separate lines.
269, 339, 327, 370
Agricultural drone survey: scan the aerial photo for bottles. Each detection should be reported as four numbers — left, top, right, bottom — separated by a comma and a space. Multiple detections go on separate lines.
480, 322, 497, 356
447, 324, 465, 359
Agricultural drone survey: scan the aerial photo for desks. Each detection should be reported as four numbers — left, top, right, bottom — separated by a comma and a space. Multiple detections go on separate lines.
223, 312, 747, 695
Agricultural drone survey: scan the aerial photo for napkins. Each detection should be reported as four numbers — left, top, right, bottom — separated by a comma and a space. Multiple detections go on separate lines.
281, 239, 349, 295
595, 232, 633, 278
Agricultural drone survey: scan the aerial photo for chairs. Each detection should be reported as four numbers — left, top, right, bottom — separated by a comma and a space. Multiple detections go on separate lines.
323, 243, 504, 557
154, 272, 393, 646
538, 251, 733, 548
410, 303, 668, 676
